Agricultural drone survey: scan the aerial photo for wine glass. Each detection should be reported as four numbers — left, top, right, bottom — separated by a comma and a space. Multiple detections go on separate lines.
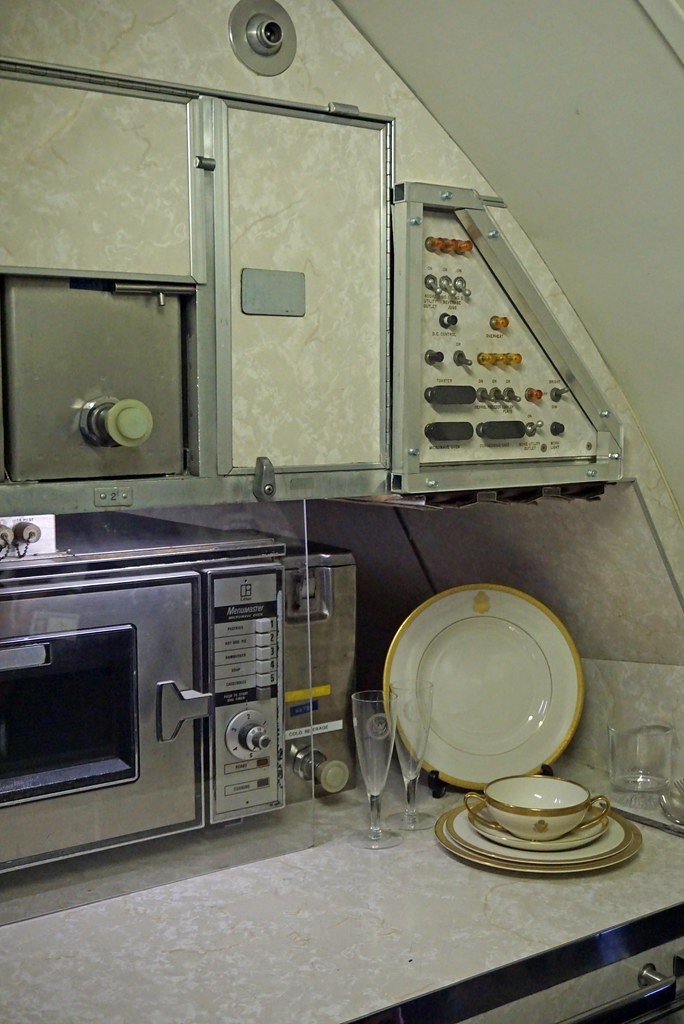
385, 676, 434, 835
346, 690, 404, 849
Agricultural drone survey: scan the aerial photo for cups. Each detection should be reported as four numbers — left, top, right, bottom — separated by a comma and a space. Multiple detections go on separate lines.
463, 775, 610, 842
607, 720, 673, 809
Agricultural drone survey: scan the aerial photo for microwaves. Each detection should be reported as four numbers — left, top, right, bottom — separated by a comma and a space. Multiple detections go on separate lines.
0, 511, 288, 876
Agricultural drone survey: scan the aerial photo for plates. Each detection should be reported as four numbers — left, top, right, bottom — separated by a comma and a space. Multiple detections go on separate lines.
434, 800, 642, 874
382, 583, 585, 791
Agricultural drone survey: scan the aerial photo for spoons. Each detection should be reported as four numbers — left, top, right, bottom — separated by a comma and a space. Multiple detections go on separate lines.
658, 790, 684, 826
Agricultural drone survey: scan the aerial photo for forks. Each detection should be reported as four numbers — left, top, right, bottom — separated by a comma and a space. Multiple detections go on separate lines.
673, 777, 684, 798
